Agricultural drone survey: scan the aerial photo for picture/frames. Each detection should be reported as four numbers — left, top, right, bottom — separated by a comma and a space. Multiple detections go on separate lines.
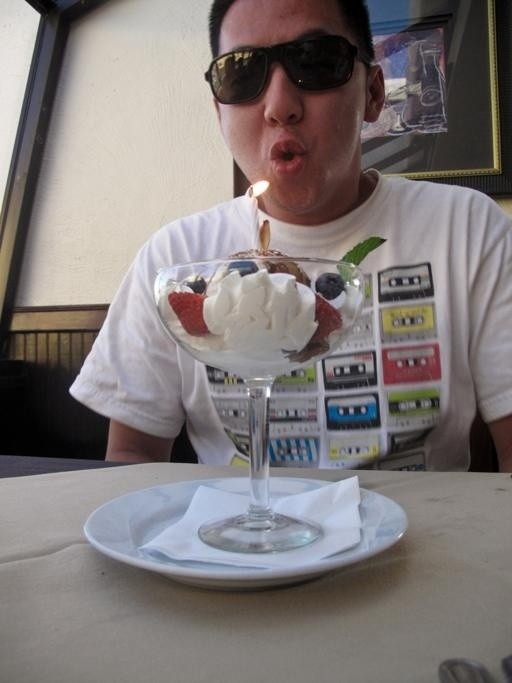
230, 0, 512, 202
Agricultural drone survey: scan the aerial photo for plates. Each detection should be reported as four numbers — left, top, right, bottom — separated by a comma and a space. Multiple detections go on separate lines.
83, 476, 409, 588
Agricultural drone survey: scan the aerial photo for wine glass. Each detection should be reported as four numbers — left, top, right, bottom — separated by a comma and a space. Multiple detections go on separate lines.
155, 252, 369, 550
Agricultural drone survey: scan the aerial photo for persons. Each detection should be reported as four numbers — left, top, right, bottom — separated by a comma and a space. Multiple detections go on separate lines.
65, 0, 510, 477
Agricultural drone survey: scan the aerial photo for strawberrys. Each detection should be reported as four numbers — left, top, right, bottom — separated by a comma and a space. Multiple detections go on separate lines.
168, 291, 209, 336
313, 294, 343, 339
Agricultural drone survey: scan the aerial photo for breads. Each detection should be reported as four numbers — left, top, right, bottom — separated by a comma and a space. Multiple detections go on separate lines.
225, 250, 311, 288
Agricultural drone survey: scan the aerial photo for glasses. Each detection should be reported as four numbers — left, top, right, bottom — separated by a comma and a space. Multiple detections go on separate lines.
203, 28, 370, 107
